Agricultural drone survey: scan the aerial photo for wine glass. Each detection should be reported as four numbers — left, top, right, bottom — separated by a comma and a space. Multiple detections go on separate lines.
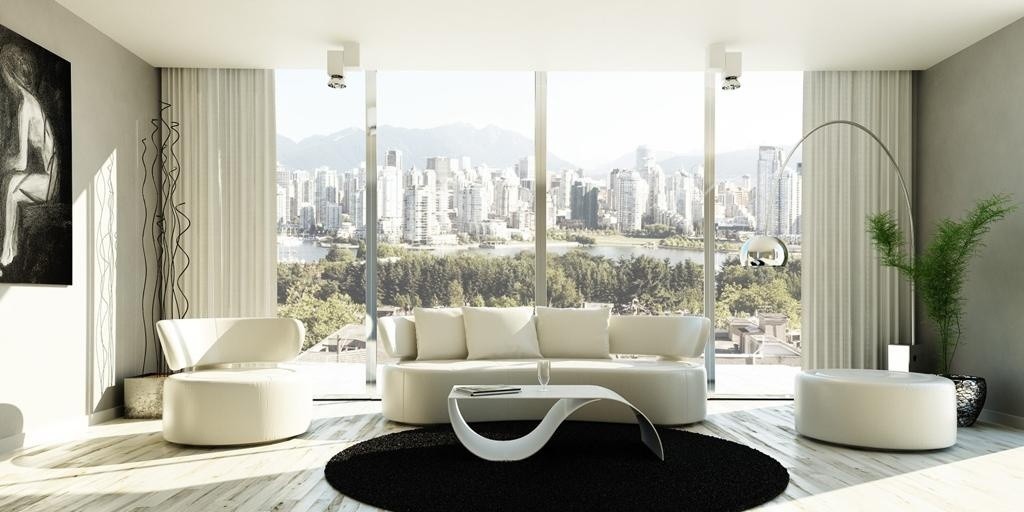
535, 359, 552, 391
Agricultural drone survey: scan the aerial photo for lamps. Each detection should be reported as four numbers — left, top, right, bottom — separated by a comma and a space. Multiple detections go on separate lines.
741, 121, 930, 374
328, 51, 348, 90
719, 53, 742, 92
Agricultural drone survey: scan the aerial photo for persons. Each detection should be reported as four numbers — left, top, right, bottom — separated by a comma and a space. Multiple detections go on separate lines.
0, 44, 61, 280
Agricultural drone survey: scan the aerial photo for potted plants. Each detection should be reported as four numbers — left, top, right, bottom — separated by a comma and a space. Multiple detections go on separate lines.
864, 192, 1024, 428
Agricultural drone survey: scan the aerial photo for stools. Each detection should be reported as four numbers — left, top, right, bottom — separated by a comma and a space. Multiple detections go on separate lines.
795, 369, 957, 450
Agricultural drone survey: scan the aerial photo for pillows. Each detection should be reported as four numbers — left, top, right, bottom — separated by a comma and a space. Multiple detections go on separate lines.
535, 304, 613, 360
463, 306, 545, 360
412, 306, 469, 360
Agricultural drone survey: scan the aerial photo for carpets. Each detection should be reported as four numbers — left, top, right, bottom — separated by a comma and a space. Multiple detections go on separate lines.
325, 416, 789, 512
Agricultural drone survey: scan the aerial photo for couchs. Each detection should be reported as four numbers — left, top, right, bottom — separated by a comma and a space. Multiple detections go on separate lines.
156, 318, 314, 446
376, 313, 711, 424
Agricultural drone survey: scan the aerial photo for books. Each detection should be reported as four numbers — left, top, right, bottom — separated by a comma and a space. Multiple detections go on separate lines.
455, 385, 522, 397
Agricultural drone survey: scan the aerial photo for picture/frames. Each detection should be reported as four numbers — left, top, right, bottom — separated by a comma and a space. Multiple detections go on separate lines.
0, 24, 72, 285
